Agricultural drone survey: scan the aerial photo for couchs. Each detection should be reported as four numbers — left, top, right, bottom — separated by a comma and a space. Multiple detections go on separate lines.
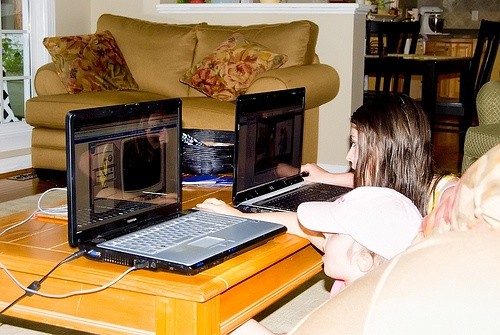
25, 14, 340, 181
461, 81, 500, 176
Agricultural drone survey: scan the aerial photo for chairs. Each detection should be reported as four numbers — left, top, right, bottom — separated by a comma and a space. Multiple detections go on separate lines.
414, 19, 500, 173
365, 20, 420, 96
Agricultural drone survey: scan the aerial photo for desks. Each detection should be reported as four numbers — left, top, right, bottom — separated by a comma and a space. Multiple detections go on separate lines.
366, 56, 473, 134
1, 173, 324, 334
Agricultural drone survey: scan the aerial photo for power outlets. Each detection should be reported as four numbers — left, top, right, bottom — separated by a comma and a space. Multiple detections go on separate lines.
472, 10, 479, 21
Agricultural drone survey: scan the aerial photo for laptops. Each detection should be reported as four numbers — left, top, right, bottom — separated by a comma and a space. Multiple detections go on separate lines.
65, 98, 288, 275
232, 87, 357, 212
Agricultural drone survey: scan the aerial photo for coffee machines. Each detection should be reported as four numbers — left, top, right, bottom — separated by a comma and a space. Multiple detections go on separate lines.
418, 6, 451, 36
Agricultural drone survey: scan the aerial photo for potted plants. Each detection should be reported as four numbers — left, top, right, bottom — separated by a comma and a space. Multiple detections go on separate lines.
377, 0, 387, 14
0, 37, 25, 117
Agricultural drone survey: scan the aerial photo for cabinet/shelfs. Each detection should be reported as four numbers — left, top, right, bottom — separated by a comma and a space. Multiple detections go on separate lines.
425, 31, 500, 131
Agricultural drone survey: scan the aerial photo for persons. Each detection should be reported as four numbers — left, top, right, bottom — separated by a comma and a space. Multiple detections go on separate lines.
192, 184, 425, 335
300, 88, 461, 218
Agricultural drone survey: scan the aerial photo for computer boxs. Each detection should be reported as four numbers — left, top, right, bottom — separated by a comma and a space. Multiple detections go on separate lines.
90, 142, 121, 215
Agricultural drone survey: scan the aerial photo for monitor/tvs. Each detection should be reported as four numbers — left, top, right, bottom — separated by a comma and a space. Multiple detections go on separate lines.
121, 133, 164, 201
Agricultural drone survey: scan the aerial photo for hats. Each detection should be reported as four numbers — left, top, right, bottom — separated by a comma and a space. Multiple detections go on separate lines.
296, 186, 423, 260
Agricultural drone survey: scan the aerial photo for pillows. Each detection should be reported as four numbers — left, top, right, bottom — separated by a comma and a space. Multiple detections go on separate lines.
180, 32, 288, 101
43, 30, 139, 95
